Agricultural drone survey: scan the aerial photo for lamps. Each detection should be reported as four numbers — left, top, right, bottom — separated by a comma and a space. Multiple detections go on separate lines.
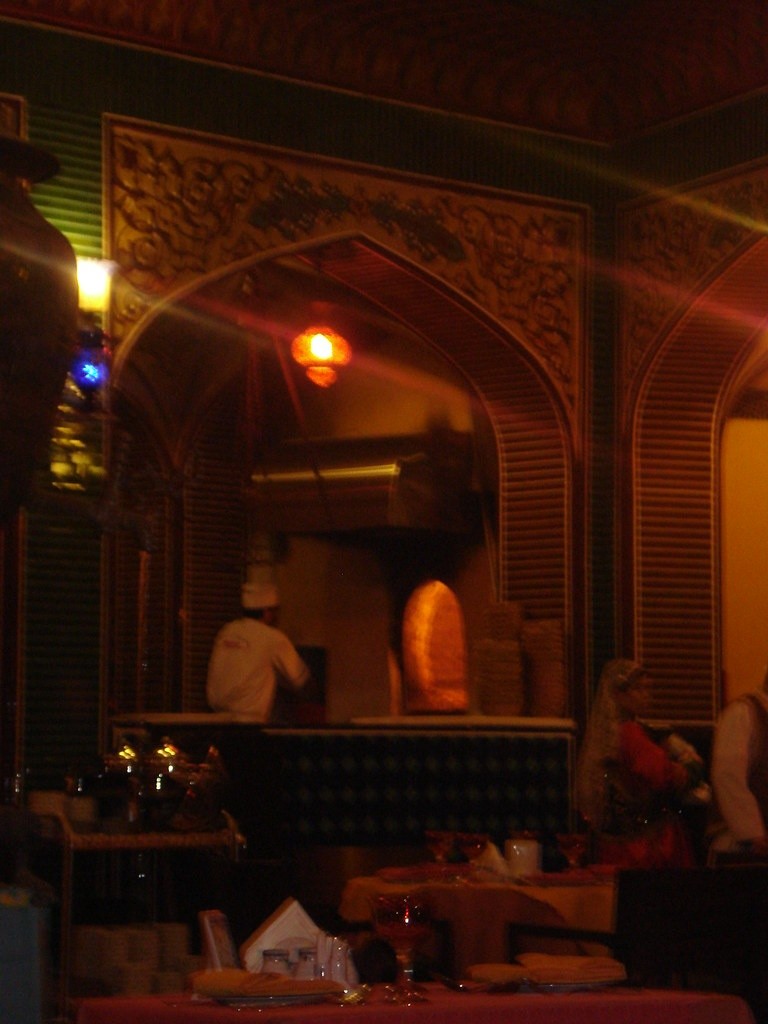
288, 265, 357, 390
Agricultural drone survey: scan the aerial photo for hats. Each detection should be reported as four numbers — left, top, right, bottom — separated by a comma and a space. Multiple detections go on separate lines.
241, 578, 280, 610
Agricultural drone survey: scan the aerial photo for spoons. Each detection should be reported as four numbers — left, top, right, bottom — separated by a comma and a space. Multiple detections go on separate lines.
428, 969, 612, 995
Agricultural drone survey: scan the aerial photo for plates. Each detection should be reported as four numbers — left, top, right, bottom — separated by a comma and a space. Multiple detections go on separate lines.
206, 992, 341, 1008
519, 983, 607, 998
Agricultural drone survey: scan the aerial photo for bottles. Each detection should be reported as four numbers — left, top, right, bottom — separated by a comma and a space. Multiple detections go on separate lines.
458, 830, 542, 874
64, 775, 86, 797
129, 853, 136, 873
110, 742, 223, 832
137, 852, 143, 875
260, 949, 319, 980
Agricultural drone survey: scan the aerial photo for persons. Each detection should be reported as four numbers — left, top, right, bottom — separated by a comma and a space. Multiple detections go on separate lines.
572, 657, 706, 988
207, 583, 311, 716
705, 672, 768, 994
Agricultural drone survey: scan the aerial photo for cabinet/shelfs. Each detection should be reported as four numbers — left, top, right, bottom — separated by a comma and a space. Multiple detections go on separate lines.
26, 809, 246, 1024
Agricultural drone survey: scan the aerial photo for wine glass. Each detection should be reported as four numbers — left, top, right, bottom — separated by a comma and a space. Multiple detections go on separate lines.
365, 872, 434, 1005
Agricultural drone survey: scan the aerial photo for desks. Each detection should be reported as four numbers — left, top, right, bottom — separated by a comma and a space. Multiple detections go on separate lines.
339, 876, 616, 990
77, 979, 757, 1024
